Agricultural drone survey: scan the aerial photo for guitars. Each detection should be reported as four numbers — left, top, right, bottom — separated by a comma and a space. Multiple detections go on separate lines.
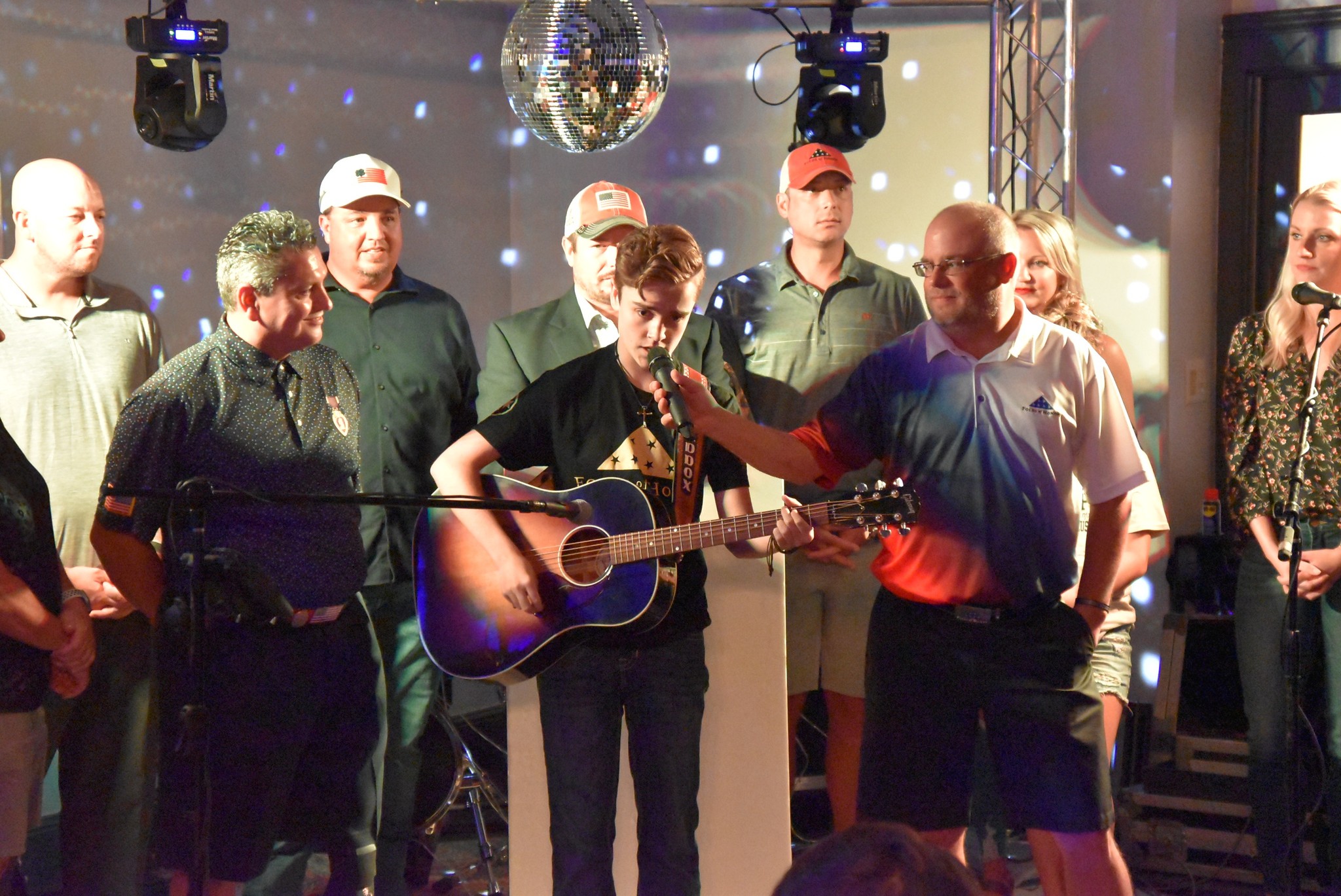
415, 472, 923, 688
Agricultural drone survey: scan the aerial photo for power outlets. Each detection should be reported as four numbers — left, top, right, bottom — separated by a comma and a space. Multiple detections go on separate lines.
1186, 362, 1216, 404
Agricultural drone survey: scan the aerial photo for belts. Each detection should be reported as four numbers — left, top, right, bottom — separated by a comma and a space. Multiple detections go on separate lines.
949, 603, 1014, 625
233, 599, 353, 626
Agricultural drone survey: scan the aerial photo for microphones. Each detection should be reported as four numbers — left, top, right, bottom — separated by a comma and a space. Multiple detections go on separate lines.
1291, 280, 1341, 308
645, 346, 694, 439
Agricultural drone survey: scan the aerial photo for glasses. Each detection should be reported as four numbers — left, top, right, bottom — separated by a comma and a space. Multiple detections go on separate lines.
912, 252, 1013, 278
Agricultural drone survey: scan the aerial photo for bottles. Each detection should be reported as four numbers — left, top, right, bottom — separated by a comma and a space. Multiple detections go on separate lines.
1202, 488, 1221, 534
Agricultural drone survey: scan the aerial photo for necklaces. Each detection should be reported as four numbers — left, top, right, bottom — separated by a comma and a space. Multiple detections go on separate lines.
615, 336, 655, 428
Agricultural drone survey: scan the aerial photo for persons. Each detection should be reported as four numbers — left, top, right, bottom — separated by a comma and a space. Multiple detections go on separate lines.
996, 209, 1153, 896
0, 328, 96, 896
243, 151, 480, 896
647, 202, 1136, 896
1, 157, 174, 895
430, 223, 817, 896
473, 179, 745, 480
709, 142, 930, 839
89, 211, 388, 895
1225, 182, 1341, 896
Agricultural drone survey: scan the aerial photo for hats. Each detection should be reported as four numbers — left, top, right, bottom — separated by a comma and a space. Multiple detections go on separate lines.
564, 179, 649, 241
779, 142, 856, 194
317, 151, 410, 213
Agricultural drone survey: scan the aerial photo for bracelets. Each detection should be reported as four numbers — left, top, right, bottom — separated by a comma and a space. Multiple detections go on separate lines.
766, 529, 800, 577
1075, 597, 1109, 614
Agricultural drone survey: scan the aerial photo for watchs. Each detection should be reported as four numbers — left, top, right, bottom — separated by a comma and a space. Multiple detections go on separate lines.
61, 589, 91, 609
